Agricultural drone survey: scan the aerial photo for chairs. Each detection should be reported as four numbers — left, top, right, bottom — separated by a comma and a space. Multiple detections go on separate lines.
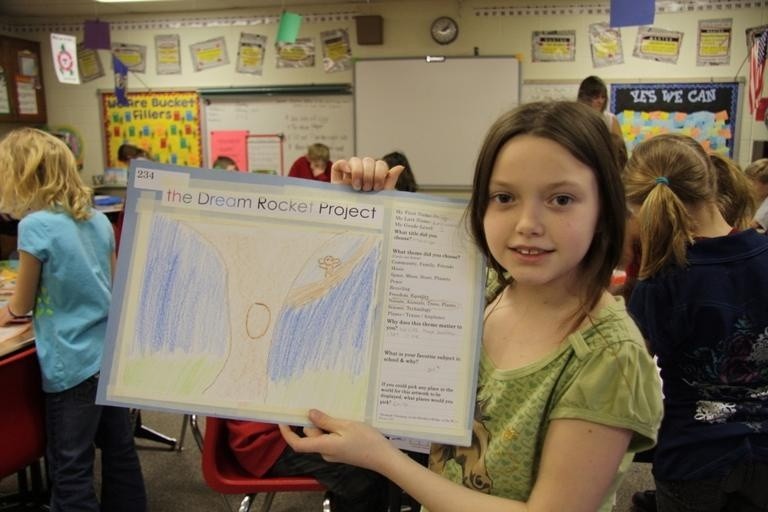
2, 346, 52, 512
203, 411, 334, 510
137, 405, 206, 458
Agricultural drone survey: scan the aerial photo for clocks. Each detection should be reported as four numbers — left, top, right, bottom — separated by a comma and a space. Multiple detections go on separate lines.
431, 16, 459, 46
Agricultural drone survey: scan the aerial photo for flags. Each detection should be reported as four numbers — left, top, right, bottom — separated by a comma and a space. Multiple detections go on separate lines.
748, 27, 768, 113
110, 55, 134, 106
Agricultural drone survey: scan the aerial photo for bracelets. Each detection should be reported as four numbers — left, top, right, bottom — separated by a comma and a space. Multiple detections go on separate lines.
6, 303, 20, 320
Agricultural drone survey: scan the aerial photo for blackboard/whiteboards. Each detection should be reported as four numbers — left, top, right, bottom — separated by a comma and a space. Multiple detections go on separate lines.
99, 91, 201, 175
520, 81, 612, 119
354, 59, 523, 190
611, 79, 744, 161
200, 91, 355, 177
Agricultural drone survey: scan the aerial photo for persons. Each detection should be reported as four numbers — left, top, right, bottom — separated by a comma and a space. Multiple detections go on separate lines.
276, 95, 667, 512
210, 156, 240, 173
615, 149, 766, 297
288, 143, 336, 183
117, 142, 156, 168
377, 152, 421, 193
224, 417, 402, 512
577, 76, 630, 168
0, 125, 149, 511
618, 131, 767, 512
